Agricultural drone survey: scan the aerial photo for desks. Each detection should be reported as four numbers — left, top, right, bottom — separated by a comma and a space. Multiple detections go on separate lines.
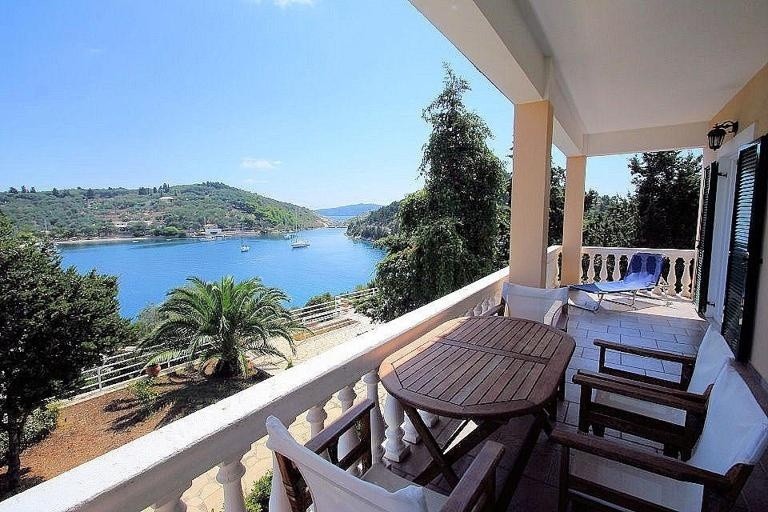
379, 316, 576, 512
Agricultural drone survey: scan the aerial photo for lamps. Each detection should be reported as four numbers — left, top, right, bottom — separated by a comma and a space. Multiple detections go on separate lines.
707, 121, 738, 151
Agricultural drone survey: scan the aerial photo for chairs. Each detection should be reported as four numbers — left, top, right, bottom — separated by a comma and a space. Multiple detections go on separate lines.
578, 326, 735, 462
265, 399, 505, 512
566, 253, 668, 311
481, 282, 569, 401
550, 362, 768, 512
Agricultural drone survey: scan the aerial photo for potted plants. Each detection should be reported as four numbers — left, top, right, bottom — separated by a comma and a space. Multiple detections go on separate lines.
146, 357, 161, 377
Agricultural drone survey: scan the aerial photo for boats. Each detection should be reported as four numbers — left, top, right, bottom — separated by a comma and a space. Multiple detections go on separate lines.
284, 233, 311, 248
199, 238, 211, 242
240, 246, 250, 252
